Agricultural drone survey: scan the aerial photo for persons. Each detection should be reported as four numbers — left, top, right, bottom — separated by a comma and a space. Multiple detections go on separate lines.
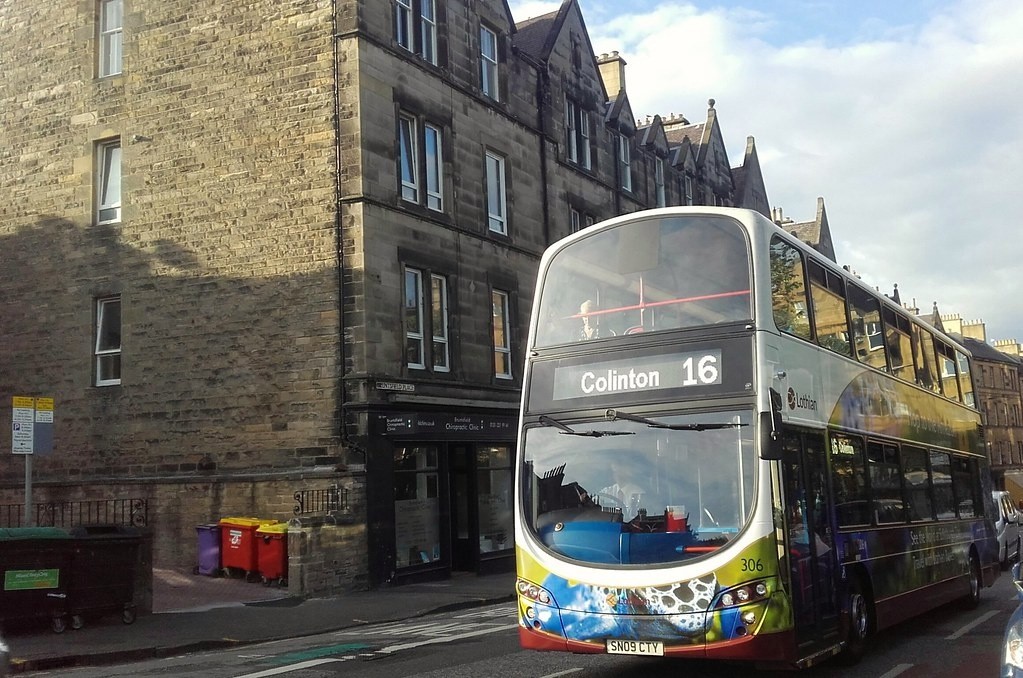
580, 460, 641, 521
569, 300, 614, 343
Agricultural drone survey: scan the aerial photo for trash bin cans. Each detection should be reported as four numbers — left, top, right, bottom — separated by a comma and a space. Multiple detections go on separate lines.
67, 523, 157, 630
255, 523, 290, 588
218, 517, 278, 583
0, 527, 68, 633
193, 524, 222, 578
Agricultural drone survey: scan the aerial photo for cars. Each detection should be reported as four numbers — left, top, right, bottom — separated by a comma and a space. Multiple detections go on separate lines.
992, 490, 1023, 568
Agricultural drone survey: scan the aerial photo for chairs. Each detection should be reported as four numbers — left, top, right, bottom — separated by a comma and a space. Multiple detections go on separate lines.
624, 324, 645, 335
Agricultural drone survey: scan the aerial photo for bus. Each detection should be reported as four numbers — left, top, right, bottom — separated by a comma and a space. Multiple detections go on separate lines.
513, 205, 1002, 671
